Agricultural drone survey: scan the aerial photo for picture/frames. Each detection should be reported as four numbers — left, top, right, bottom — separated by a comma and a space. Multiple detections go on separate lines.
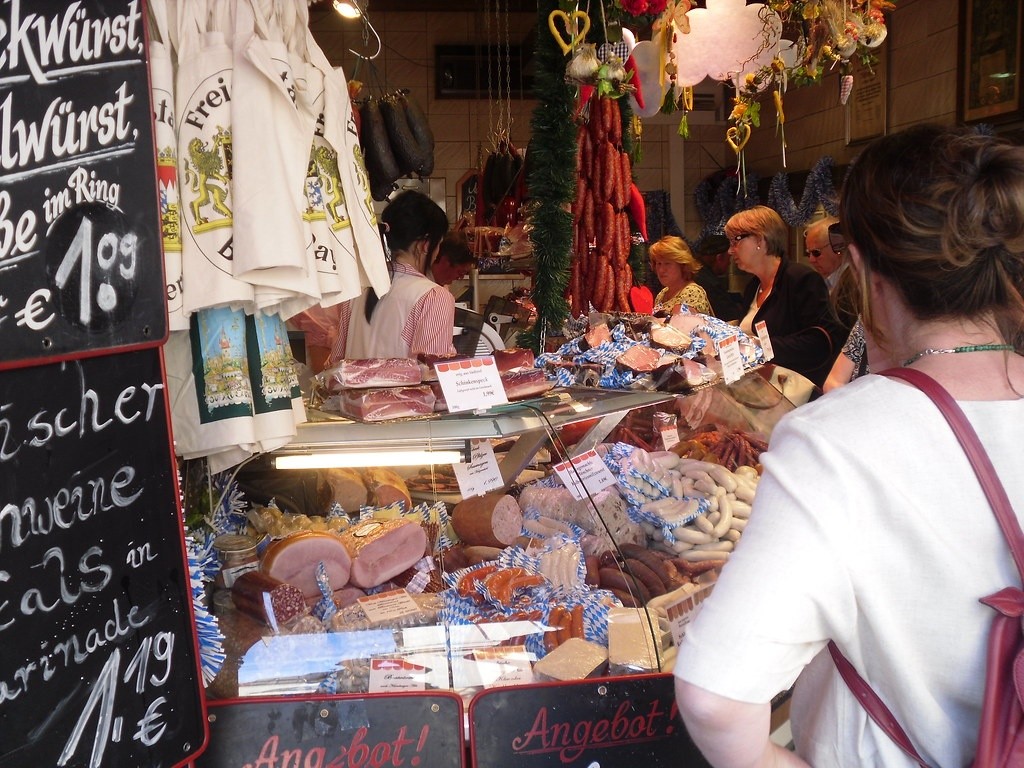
956, 0, 1024, 128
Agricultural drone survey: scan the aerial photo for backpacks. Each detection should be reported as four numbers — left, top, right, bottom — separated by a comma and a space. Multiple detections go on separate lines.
827, 367, 1024, 768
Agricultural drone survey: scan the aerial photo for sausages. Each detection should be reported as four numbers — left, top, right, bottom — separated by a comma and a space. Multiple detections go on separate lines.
569, 88, 634, 319
348, 88, 433, 203
454, 413, 771, 663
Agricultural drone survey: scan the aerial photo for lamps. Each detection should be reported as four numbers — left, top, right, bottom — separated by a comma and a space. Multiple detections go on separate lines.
268, 443, 472, 470
332, 0, 366, 20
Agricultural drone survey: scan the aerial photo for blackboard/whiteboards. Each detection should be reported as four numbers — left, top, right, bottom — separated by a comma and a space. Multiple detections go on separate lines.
456, 168, 479, 224
0, 0, 170, 371
468, 672, 714, 768
0, 346, 210, 768
188, 692, 469, 768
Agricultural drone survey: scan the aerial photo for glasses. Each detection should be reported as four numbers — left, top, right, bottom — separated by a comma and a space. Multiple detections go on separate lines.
729, 233, 751, 247
804, 242, 830, 258
828, 223, 897, 279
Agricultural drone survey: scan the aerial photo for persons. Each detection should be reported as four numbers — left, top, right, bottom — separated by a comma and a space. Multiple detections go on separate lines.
673, 120, 1024, 768
649, 235, 714, 317
723, 206, 832, 408
320, 189, 457, 370
824, 314, 871, 393
425, 231, 473, 286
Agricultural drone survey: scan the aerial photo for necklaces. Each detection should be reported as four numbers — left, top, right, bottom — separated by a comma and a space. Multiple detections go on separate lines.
902, 343, 1015, 367
803, 217, 863, 332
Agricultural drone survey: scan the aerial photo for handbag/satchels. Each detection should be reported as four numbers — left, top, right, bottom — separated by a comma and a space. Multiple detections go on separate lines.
686, 363, 823, 444
145, 0, 392, 475
